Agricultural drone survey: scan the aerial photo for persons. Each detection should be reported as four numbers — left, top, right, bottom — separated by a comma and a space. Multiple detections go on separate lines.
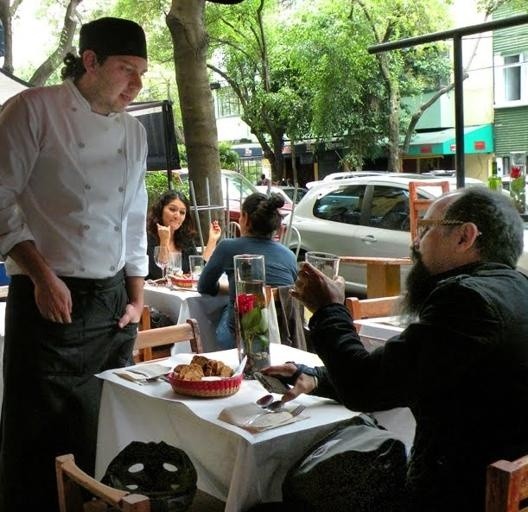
259, 186, 528, 512
196, 191, 299, 348
144, 190, 222, 328
0, 16, 150, 511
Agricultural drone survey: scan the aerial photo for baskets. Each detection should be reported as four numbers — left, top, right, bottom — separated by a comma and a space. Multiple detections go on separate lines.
169, 274, 195, 287
162, 373, 243, 398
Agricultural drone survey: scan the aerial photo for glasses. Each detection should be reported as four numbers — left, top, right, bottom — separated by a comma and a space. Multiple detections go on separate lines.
414, 216, 482, 237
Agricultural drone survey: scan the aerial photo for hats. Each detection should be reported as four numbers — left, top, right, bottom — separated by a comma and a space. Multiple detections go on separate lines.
80, 19, 147, 60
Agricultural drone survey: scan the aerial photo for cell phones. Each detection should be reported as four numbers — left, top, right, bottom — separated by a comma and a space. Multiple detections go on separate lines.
254, 372, 290, 394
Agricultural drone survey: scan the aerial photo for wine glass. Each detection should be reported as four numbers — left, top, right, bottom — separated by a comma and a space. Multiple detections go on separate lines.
154, 245, 169, 281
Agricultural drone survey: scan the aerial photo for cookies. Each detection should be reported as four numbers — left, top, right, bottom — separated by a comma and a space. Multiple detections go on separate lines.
170, 354, 234, 382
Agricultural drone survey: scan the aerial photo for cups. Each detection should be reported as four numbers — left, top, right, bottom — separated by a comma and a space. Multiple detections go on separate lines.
301, 250, 341, 332
166, 252, 205, 292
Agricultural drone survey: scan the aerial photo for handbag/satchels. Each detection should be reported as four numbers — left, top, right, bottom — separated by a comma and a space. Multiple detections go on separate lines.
282, 411, 407, 512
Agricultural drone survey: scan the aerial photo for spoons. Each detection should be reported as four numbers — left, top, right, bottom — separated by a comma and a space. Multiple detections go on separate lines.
256, 395, 275, 407
126, 368, 159, 382
265, 400, 283, 413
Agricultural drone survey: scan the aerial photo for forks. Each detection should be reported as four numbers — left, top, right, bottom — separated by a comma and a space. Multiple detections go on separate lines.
291, 404, 306, 416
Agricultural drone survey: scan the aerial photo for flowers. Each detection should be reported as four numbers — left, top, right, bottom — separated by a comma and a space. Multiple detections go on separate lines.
512, 167, 526, 212
237, 294, 267, 371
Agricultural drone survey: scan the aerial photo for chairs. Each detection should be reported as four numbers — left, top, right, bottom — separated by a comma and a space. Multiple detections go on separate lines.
187, 176, 301, 260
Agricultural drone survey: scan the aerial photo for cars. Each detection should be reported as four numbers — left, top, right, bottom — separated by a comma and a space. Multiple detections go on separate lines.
170, 168, 262, 238
324, 170, 519, 209
282, 176, 457, 300
254, 184, 309, 219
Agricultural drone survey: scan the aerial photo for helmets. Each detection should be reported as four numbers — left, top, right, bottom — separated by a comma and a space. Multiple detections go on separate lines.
98, 441, 197, 510
